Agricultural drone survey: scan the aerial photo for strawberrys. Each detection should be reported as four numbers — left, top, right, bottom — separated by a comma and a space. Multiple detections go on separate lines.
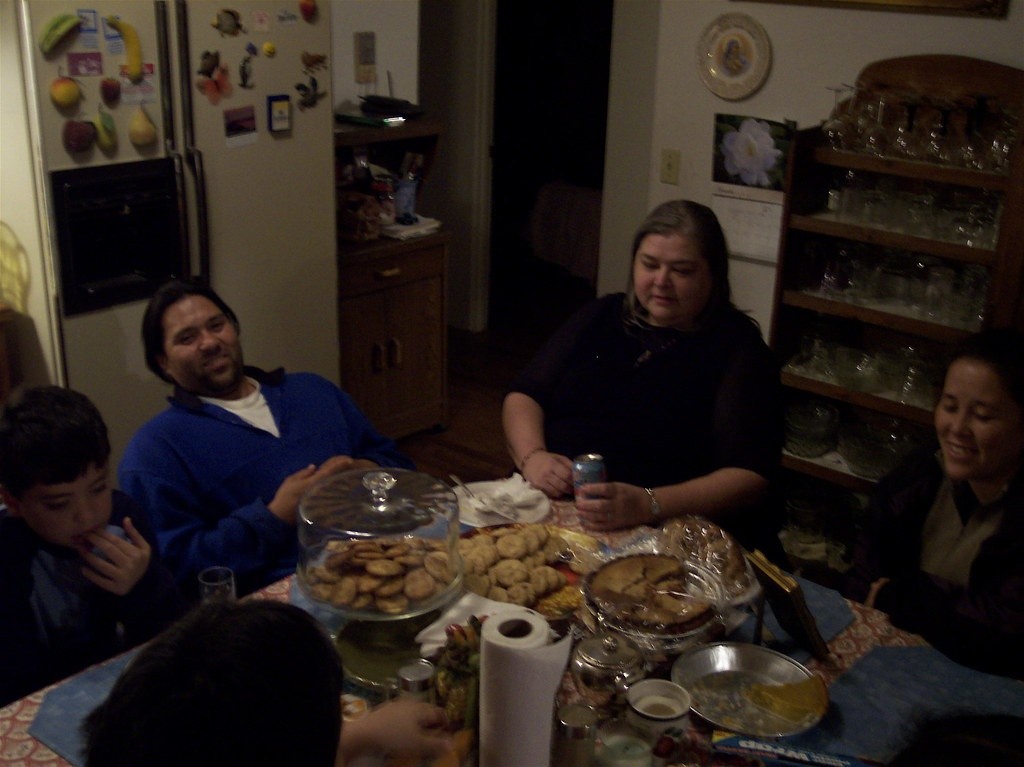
100, 78, 121, 103
300, 0, 318, 22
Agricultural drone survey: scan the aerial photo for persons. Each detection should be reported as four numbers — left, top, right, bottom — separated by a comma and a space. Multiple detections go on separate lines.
84, 601, 453, 767
501, 199, 786, 563
842, 327, 1024, 678
114, 280, 416, 600
0, 386, 188, 709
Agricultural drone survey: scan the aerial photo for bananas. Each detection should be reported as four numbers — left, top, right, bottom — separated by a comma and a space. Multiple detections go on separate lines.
101, 15, 142, 82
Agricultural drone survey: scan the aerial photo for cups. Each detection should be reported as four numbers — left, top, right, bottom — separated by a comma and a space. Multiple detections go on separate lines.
200, 566, 235, 606
626, 679, 690, 754
597, 715, 660, 767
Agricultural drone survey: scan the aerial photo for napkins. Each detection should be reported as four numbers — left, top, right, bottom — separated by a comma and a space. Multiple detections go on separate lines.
415, 592, 561, 658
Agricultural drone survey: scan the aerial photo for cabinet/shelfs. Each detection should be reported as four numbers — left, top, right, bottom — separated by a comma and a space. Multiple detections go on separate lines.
768, 53, 1023, 520
334, 114, 454, 443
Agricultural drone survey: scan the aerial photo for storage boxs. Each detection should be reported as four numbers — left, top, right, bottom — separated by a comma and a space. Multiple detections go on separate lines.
712, 730, 872, 767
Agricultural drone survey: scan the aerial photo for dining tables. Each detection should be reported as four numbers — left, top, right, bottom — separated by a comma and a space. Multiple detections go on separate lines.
0, 492, 1023, 767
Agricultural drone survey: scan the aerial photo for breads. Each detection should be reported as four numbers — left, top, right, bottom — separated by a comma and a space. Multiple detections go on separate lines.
585, 516, 748, 633
746, 674, 830, 724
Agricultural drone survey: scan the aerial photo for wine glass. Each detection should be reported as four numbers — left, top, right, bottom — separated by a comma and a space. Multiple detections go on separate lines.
790, 80, 1019, 414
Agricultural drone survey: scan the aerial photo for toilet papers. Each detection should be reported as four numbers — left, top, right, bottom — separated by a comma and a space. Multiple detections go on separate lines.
479, 609, 573, 767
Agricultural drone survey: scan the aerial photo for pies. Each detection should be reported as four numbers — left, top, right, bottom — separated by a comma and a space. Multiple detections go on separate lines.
453, 525, 602, 620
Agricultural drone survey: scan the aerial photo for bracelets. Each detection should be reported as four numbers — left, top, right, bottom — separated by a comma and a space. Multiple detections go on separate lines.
519, 448, 543, 476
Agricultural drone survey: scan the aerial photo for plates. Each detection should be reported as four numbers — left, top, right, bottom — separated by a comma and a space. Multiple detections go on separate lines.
584, 551, 724, 641
672, 642, 828, 737
445, 480, 552, 531
449, 518, 606, 618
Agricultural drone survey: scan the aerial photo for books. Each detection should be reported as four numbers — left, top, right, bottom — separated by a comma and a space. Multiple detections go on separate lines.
380, 213, 443, 240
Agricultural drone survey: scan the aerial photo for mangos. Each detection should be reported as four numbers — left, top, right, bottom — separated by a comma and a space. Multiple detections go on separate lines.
93, 114, 119, 151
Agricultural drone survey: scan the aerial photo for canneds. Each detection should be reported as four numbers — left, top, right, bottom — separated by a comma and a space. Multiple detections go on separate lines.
572, 454, 604, 496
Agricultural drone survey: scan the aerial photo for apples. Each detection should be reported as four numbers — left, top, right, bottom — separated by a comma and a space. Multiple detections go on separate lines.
63, 121, 97, 150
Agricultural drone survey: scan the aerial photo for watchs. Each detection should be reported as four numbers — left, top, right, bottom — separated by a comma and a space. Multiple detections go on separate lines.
644, 487, 665, 528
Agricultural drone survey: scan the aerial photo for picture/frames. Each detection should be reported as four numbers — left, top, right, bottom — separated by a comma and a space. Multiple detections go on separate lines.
746, 552, 828, 657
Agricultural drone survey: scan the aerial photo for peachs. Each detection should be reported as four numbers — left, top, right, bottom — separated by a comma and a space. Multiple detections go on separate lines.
51, 77, 79, 105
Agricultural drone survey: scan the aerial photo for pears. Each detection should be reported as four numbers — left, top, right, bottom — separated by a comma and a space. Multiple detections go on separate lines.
129, 110, 157, 146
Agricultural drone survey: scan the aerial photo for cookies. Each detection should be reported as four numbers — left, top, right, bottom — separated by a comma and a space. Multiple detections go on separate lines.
296, 538, 456, 612
